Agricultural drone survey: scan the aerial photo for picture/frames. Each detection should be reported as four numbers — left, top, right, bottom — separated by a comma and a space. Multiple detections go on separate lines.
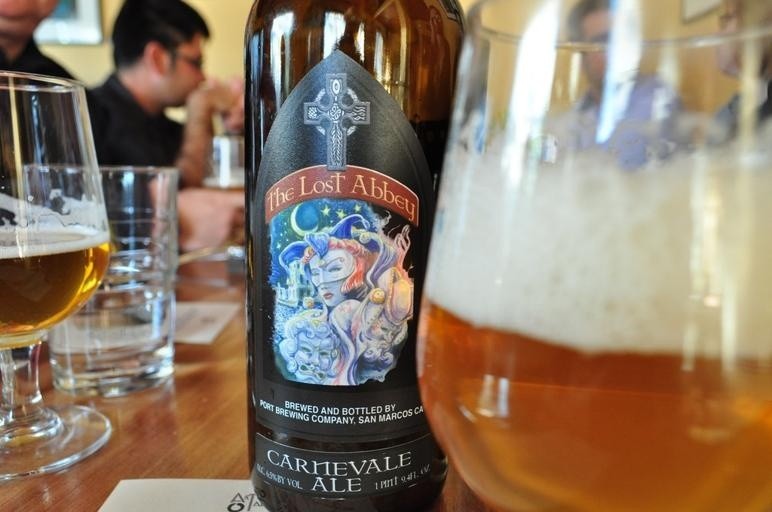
31, 0, 104, 47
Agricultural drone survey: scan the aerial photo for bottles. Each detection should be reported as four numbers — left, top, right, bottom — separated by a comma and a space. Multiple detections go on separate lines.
240, 1, 465, 512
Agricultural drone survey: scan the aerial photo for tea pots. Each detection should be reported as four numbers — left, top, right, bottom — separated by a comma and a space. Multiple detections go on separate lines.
201, 136, 244, 188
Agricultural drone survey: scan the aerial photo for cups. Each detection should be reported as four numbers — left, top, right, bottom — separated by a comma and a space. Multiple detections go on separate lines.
418, 5, 772, 510
20, 161, 180, 399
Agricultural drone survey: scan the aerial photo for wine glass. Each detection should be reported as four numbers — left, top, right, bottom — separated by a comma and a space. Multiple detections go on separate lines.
1, 70, 114, 483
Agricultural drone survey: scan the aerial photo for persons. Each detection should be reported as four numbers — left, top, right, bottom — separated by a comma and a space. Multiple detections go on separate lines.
91, 1, 245, 187
553, 1, 685, 169
353, 266, 416, 370
1, 1, 248, 254
278, 213, 411, 385
708, 0, 772, 144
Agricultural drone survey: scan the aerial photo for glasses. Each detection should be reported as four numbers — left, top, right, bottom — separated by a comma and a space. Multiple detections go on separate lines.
176, 54, 202, 70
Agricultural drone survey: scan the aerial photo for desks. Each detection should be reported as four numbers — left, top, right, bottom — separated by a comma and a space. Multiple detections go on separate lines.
1, 249, 485, 511
